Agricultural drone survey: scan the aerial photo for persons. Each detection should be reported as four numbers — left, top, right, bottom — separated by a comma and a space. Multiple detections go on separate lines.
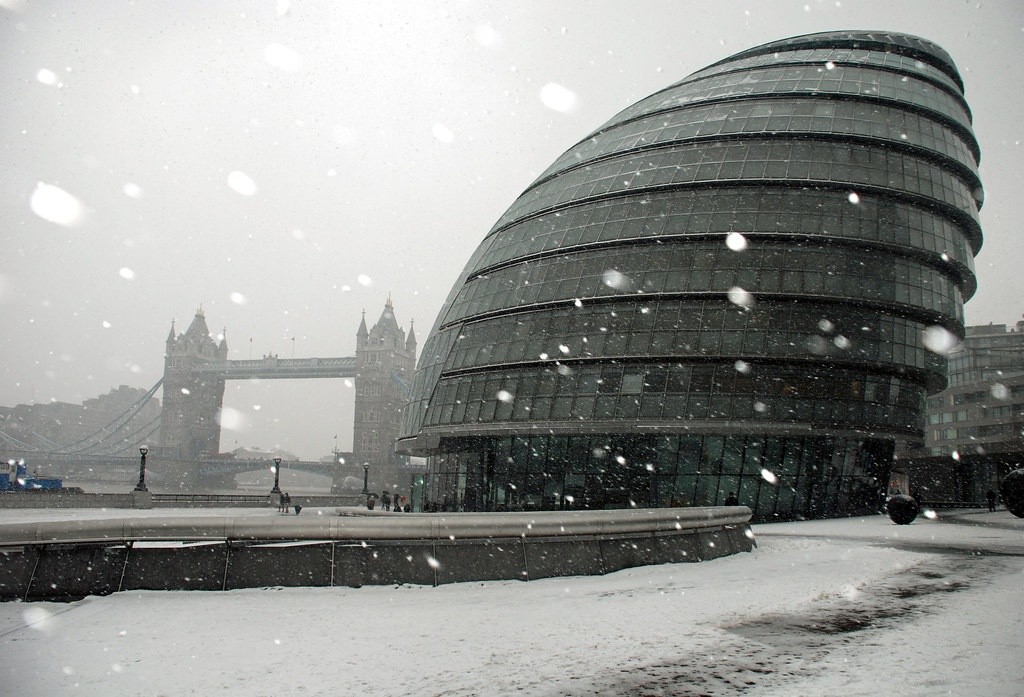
277, 495, 284, 512
381, 495, 387, 510
404, 504, 412, 512
385, 498, 391, 511
422, 499, 507, 512
393, 502, 402, 512
725, 492, 739, 506
986, 488, 996, 511
393, 494, 399, 507
284, 493, 290, 513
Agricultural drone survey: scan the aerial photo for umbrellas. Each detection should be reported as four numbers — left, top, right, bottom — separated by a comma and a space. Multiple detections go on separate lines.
383, 491, 390, 495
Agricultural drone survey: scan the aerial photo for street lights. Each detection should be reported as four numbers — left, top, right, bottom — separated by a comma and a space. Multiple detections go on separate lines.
134, 445, 149, 492
361, 462, 370, 494
271, 457, 281, 493
